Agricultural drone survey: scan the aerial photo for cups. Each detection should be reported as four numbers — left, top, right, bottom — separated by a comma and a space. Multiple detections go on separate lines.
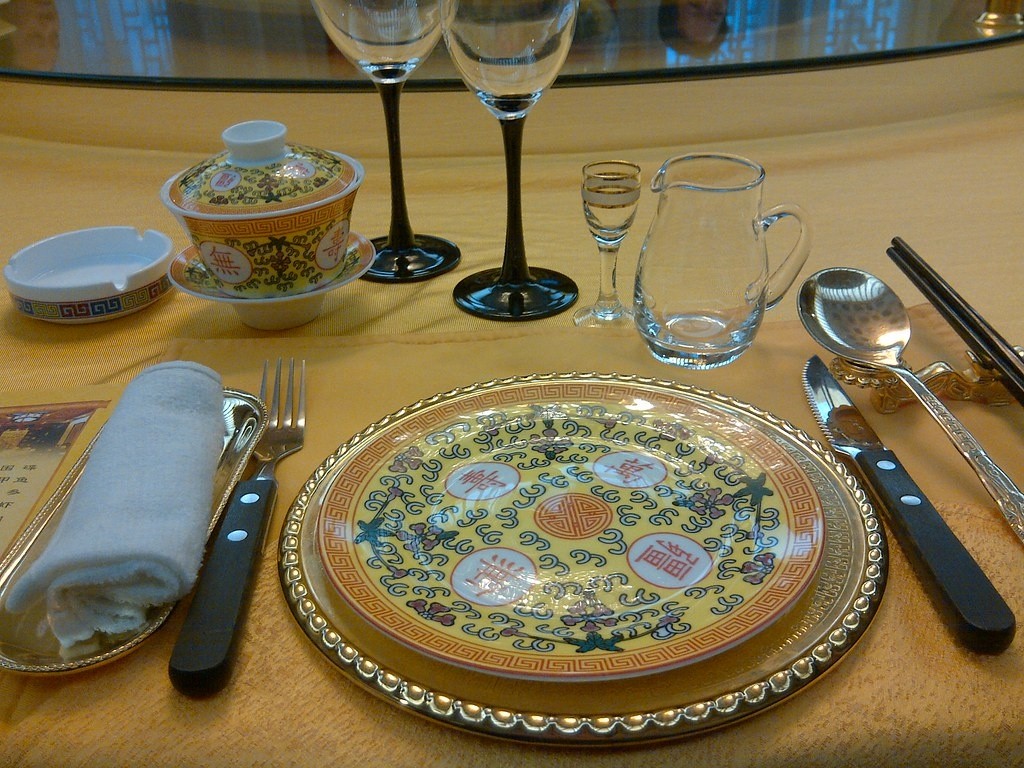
972, 0, 1024, 28
157, 118, 366, 301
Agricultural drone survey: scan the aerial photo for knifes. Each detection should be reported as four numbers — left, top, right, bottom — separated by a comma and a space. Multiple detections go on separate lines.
799, 352, 1018, 659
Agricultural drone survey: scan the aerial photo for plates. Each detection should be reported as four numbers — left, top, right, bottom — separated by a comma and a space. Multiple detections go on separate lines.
277, 372, 892, 747
166, 229, 377, 331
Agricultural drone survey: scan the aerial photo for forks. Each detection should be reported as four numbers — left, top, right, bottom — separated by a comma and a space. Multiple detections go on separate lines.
167, 354, 310, 700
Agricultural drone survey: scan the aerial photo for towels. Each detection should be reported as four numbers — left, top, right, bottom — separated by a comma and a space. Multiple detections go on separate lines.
7, 361, 224, 658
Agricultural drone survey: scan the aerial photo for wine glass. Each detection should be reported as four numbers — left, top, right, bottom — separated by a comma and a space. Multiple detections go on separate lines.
438, 0, 581, 322
572, 159, 643, 328
309, 0, 463, 283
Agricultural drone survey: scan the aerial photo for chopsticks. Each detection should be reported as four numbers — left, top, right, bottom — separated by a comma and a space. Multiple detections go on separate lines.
883, 235, 1024, 403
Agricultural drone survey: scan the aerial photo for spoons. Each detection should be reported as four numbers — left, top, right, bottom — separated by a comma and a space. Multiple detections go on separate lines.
796, 267, 1024, 543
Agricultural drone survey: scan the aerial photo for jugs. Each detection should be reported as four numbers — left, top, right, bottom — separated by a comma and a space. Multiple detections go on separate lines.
631, 148, 814, 369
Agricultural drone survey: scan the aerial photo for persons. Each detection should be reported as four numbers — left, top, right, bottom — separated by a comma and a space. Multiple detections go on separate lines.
657, 0, 731, 57
0, 0, 61, 72
